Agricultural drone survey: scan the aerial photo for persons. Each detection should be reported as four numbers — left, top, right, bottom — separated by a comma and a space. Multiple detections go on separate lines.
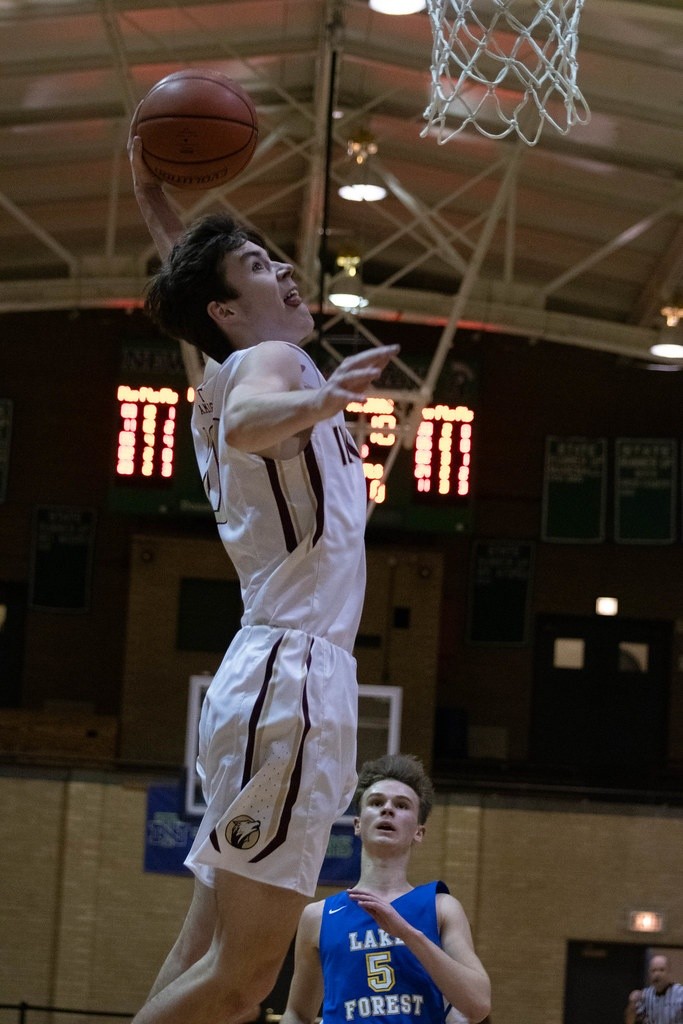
280, 755, 491, 1024
128, 98, 401, 1024
628, 955, 683, 1024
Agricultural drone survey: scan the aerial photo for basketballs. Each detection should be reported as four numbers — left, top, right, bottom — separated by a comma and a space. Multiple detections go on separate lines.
130, 68, 261, 190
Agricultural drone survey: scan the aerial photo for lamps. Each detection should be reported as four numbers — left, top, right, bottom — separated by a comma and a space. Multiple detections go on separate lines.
649, 301, 683, 358
328, 243, 369, 308
338, 110, 387, 201
